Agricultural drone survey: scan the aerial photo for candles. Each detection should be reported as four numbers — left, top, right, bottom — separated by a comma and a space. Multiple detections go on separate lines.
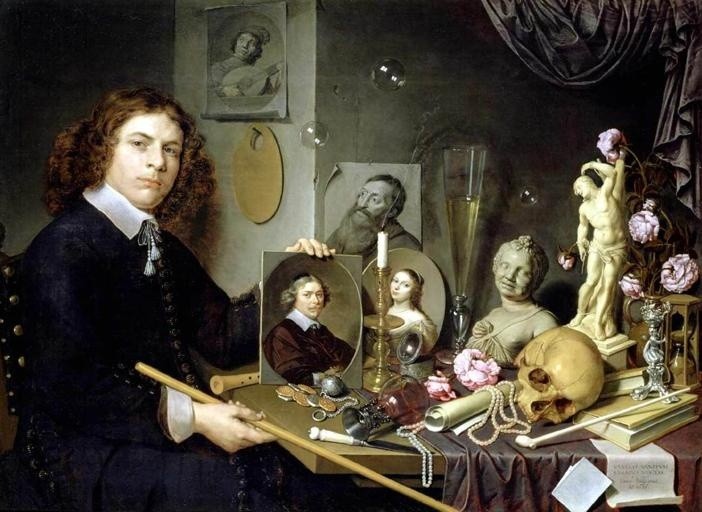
377, 231, 389, 269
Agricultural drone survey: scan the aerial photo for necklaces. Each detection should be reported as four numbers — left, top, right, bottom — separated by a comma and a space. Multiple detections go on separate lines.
395, 380, 532, 488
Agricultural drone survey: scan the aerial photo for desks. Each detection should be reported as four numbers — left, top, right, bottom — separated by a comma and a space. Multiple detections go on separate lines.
199, 360, 697, 490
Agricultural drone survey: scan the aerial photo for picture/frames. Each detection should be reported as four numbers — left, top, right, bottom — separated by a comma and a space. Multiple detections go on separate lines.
257, 249, 365, 390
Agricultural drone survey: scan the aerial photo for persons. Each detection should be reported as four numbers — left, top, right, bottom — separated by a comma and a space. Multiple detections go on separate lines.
263, 273, 355, 387
209, 25, 282, 96
647, 328, 666, 363
325, 175, 423, 272
385, 269, 438, 354
7, 86, 335, 512
569, 161, 627, 343
465, 235, 560, 370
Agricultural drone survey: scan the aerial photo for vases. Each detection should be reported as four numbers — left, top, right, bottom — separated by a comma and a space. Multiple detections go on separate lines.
629, 298, 678, 404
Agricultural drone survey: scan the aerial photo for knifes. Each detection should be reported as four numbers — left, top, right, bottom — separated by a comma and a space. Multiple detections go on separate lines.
307, 427, 435, 455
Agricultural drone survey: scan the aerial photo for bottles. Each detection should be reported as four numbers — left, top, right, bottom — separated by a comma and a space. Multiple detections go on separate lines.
341, 376, 432, 441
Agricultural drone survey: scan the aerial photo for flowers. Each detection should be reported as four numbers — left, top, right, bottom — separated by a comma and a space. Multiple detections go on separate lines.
451, 348, 501, 388
557, 124, 700, 304
425, 373, 459, 404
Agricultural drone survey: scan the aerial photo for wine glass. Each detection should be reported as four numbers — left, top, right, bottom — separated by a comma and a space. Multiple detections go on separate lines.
435, 147, 487, 366
